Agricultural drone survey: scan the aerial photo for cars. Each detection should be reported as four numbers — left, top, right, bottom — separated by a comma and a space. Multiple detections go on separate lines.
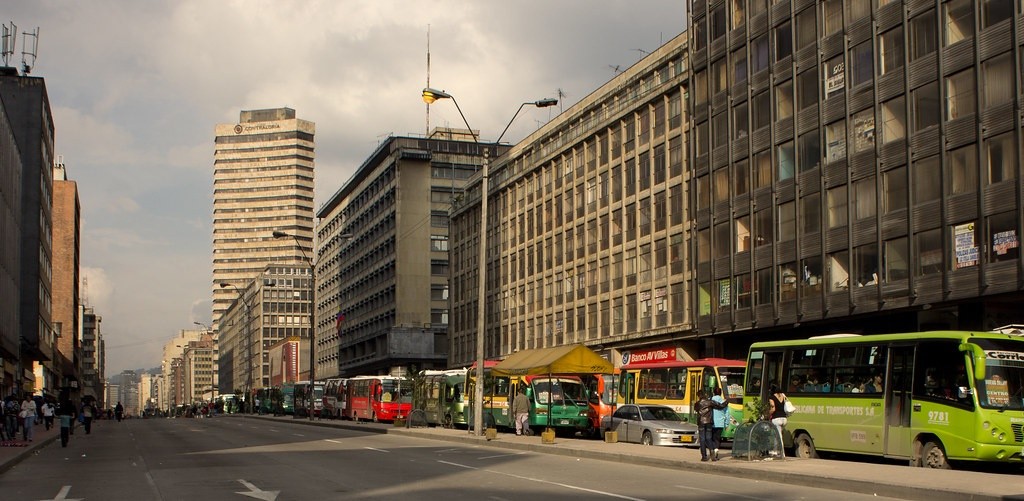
145, 407, 160, 418
600, 404, 700, 449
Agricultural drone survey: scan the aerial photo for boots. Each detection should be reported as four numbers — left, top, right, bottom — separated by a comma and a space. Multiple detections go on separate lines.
701, 452, 708, 461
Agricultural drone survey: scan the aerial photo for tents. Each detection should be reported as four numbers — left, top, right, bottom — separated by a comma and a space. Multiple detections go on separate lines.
490, 344, 620, 432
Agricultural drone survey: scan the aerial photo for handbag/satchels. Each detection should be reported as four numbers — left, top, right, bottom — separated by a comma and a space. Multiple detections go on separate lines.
18, 410, 28, 418
782, 393, 797, 415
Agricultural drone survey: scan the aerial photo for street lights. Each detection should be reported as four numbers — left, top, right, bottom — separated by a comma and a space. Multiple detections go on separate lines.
272, 231, 354, 421
219, 282, 278, 389
193, 321, 215, 409
176, 345, 192, 406
421, 86, 559, 442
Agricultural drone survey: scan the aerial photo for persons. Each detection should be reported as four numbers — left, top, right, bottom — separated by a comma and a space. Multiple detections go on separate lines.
767, 382, 788, 458
252, 397, 259, 414
115, 402, 124, 422
192, 405, 207, 419
993, 375, 1000, 380
512, 388, 533, 435
790, 369, 883, 392
107, 409, 112, 420
711, 387, 730, 459
694, 390, 729, 461
182, 403, 186, 412
750, 377, 760, 393
672, 386, 683, 396
227, 400, 232, 414
238, 399, 245, 413
0, 391, 96, 447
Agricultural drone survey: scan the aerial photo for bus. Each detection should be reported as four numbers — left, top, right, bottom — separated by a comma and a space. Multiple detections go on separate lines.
245, 386, 272, 414
618, 358, 763, 441
345, 374, 416, 425
322, 378, 349, 422
272, 383, 294, 415
474, 359, 623, 433
292, 381, 326, 416
210, 393, 241, 414
417, 367, 470, 430
738, 324, 1024, 471
462, 366, 589, 436
